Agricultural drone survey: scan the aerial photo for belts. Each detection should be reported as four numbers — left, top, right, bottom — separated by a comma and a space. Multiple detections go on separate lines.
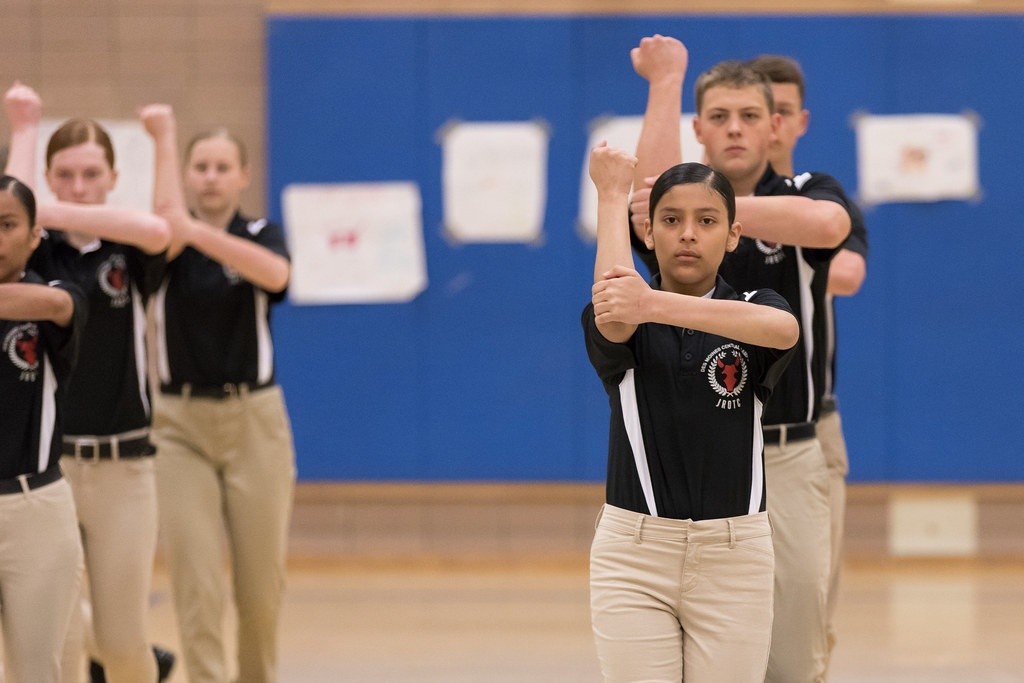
0, 466, 61, 494
819, 395, 836, 414
59, 434, 157, 458
160, 376, 278, 399
763, 425, 817, 446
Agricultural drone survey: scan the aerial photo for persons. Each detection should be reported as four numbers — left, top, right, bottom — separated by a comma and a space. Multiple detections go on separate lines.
628, 33, 852, 683
140, 104, 296, 683
4, 79, 172, 683
0, 176, 90, 683
582, 140, 799, 683
704, 54, 866, 683
